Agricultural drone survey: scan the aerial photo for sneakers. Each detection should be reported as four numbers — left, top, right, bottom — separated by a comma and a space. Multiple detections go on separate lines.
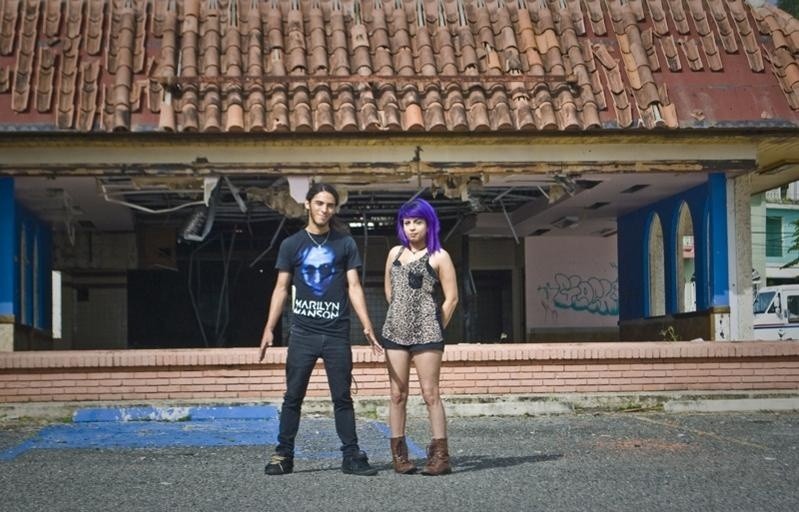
265, 455, 294, 475
343, 454, 378, 476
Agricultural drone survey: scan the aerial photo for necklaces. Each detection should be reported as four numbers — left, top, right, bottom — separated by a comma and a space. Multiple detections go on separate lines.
302, 228, 331, 248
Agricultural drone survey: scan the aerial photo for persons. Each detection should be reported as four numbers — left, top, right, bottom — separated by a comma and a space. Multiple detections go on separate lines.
297, 244, 336, 297
380, 198, 459, 476
256, 181, 384, 475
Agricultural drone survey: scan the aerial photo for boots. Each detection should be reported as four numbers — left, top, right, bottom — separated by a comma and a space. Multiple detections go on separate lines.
422, 437, 450, 475
387, 435, 416, 475
